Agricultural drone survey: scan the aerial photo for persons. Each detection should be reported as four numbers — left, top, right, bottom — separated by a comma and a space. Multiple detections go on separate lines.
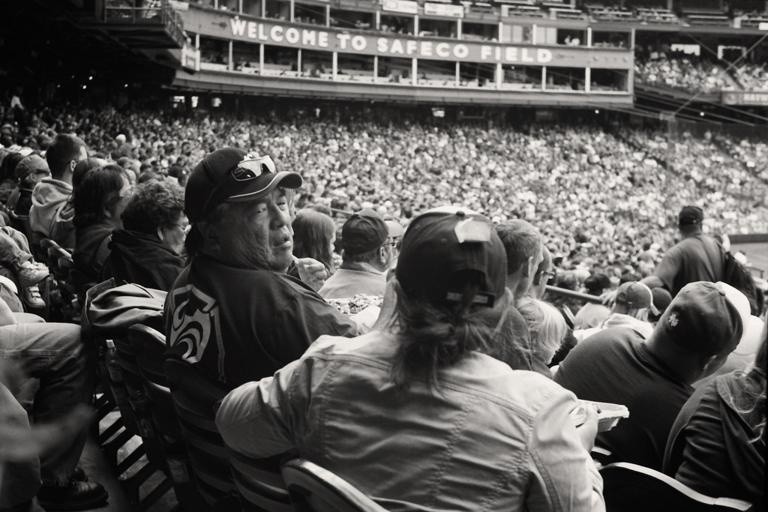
2, 2, 768, 512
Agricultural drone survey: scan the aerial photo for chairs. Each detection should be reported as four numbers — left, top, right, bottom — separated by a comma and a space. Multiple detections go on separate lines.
137, 377, 219, 511
594, 461, 751, 510
225, 448, 293, 511
279, 459, 392, 511
106, 323, 171, 443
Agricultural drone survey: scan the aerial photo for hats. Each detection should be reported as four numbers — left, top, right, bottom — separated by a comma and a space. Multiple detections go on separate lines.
616, 282, 653, 307
185, 148, 302, 223
397, 212, 509, 306
662, 281, 742, 350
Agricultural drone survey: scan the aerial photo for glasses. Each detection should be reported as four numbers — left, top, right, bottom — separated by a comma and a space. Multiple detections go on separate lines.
231, 155, 276, 181
